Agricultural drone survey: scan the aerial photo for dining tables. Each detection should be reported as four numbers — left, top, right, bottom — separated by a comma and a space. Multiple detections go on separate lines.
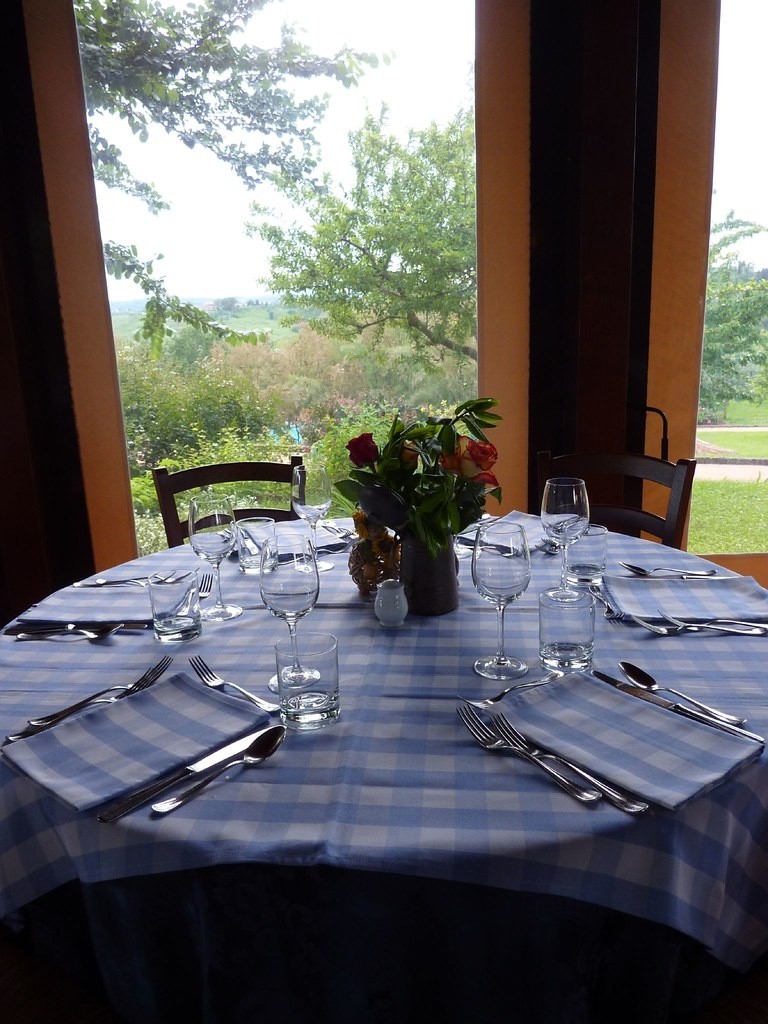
0, 519, 768, 1023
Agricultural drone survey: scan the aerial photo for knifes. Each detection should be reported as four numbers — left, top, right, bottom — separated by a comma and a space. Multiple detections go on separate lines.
96, 725, 288, 825
620, 613, 767, 623
618, 574, 734, 580
590, 669, 766, 746
241, 528, 258, 556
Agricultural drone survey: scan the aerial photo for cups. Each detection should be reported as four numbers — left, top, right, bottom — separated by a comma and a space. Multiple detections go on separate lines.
560, 524, 608, 592
273, 632, 342, 733
147, 568, 203, 643
538, 587, 597, 674
235, 516, 279, 576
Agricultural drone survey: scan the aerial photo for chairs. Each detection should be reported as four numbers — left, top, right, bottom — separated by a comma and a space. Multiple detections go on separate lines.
151, 455, 303, 547
536, 447, 698, 550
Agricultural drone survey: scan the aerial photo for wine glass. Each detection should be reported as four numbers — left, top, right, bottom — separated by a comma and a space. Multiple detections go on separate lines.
471, 520, 531, 681
291, 465, 335, 573
541, 476, 590, 602
187, 493, 243, 622
259, 533, 322, 690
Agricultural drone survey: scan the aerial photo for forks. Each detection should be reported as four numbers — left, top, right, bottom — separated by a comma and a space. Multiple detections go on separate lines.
95, 571, 177, 586
26, 655, 174, 727
5, 666, 161, 743
631, 613, 766, 636
588, 585, 625, 620
456, 704, 603, 804
73, 571, 193, 588
456, 670, 564, 710
657, 609, 768, 632
199, 573, 213, 598
322, 526, 353, 538
534, 538, 579, 555
542, 521, 590, 547
489, 712, 651, 815
277, 539, 356, 566
188, 655, 281, 712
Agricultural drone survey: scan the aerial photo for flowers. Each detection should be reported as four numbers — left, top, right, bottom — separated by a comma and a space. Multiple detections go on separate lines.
332, 395, 503, 559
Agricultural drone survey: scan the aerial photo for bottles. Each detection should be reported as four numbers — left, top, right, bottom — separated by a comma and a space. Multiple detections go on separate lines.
374, 579, 409, 629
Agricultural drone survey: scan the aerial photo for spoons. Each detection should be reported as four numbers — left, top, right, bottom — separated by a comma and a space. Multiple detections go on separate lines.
151, 726, 286, 815
619, 561, 717, 576
16, 623, 124, 641
618, 661, 747, 726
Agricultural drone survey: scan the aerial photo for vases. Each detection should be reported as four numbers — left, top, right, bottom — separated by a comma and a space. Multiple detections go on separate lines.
389, 524, 461, 618
